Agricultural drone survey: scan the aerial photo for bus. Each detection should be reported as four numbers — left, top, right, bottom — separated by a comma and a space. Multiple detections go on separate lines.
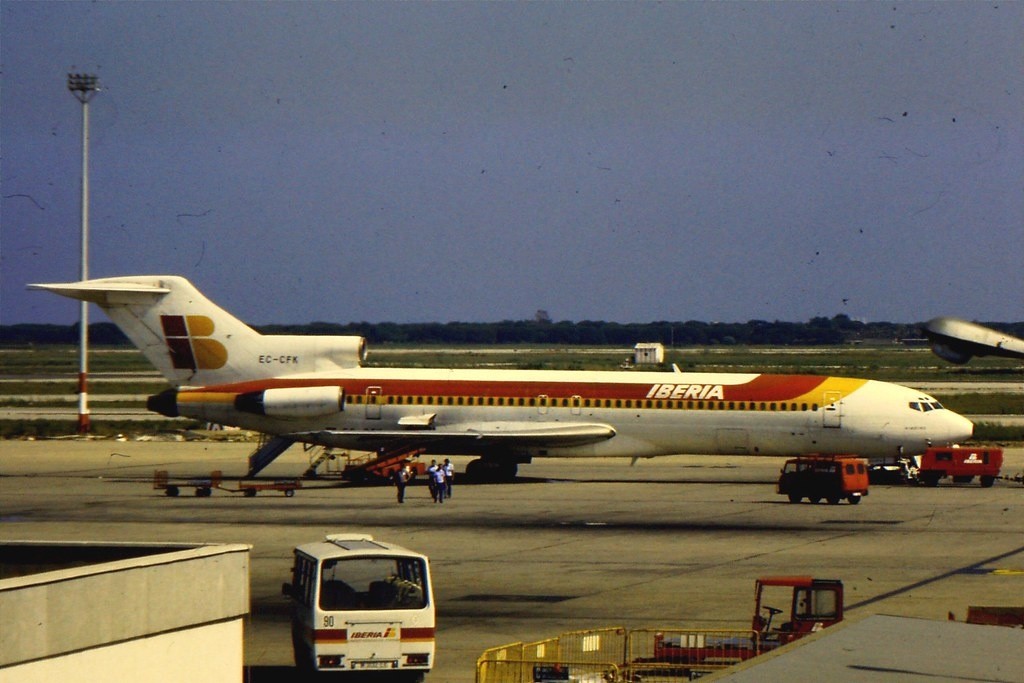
278, 533, 436, 683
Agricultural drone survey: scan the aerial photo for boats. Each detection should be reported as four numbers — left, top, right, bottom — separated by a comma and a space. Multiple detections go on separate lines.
25, 272, 976, 482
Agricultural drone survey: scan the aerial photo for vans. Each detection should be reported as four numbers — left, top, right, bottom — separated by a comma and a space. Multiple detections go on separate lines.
776, 455, 868, 504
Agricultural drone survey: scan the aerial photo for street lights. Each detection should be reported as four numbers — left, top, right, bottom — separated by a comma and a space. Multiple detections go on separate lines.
66, 70, 102, 433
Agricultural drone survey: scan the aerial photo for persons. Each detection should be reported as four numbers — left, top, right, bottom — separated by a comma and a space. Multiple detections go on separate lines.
394, 463, 407, 503
427, 459, 455, 503
